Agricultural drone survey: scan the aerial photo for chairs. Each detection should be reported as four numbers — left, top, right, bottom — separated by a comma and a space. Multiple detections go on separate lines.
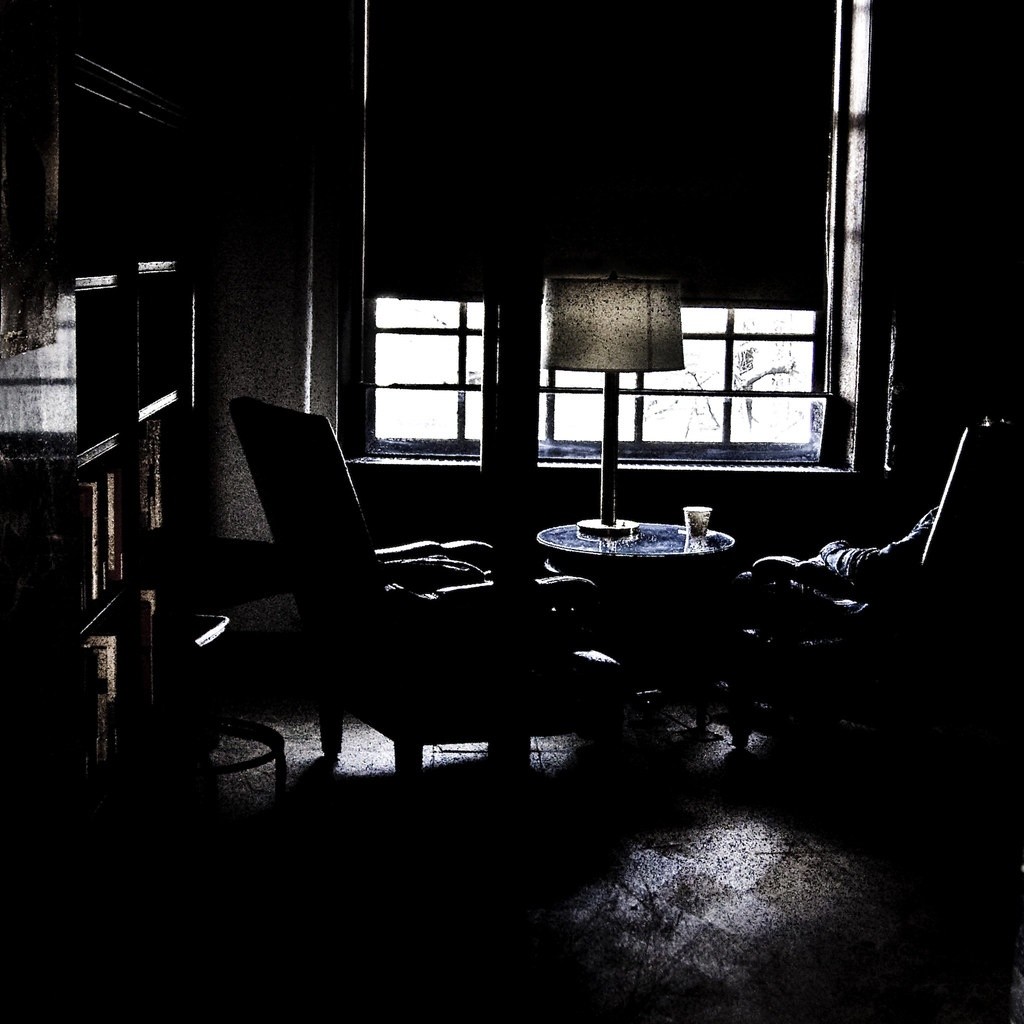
721, 419, 1024, 756
227, 392, 604, 807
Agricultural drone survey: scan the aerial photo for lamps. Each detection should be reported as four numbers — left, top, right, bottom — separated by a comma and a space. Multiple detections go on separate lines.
541, 269, 683, 541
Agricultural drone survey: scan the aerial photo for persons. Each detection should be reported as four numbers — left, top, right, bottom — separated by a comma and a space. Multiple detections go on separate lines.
748, 416, 1019, 600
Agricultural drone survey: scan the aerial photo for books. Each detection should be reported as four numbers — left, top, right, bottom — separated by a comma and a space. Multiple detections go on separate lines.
79, 415, 165, 777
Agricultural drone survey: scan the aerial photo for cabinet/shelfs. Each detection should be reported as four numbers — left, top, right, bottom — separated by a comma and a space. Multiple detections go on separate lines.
138, 261, 194, 774
0, 272, 139, 839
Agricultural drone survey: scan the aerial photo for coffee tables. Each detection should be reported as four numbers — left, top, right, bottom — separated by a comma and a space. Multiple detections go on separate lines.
534, 517, 740, 745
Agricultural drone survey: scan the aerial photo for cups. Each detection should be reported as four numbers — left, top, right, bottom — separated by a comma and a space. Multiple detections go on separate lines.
682, 506, 713, 538
